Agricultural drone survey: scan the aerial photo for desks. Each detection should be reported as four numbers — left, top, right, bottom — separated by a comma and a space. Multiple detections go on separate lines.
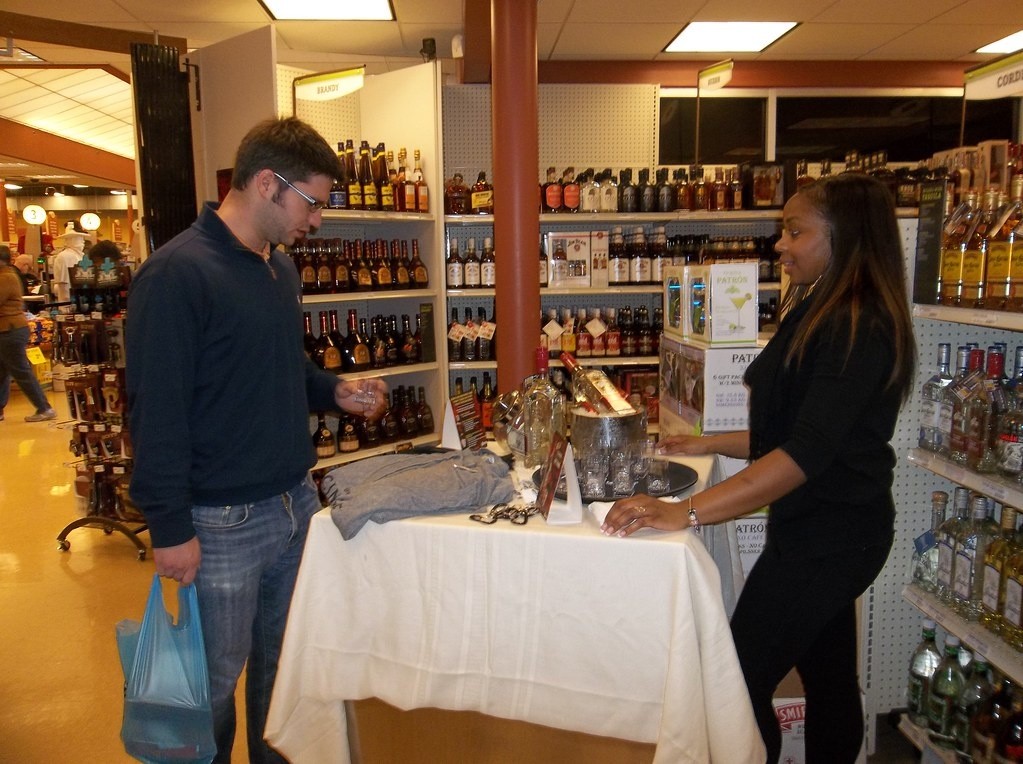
260, 457, 768, 764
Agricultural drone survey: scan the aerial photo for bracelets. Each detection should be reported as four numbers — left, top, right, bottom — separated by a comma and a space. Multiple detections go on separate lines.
689, 497, 699, 529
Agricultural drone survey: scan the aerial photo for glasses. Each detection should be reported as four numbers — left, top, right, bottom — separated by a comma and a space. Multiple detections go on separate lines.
274, 173, 324, 213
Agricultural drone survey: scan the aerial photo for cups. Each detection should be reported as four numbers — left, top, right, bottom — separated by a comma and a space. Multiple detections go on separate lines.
555, 437, 671, 497
354, 374, 380, 404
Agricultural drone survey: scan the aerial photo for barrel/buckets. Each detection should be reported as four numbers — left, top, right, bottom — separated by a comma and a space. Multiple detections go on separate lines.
569, 403, 648, 451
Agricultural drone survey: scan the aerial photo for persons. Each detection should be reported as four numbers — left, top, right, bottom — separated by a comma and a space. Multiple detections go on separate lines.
15, 254, 44, 314
124, 117, 386, 764
0, 245, 57, 422
600, 175, 914, 764
55, 235, 85, 314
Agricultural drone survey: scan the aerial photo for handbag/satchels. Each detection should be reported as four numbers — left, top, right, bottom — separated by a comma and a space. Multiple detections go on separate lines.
116, 575, 218, 764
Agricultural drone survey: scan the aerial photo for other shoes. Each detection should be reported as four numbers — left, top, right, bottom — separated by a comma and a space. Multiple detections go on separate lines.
0, 409, 4, 420
24, 409, 56, 422
250, 743, 290, 764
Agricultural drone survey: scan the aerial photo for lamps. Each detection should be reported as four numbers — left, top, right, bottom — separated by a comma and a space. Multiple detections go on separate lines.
79, 211, 101, 232
23, 204, 46, 225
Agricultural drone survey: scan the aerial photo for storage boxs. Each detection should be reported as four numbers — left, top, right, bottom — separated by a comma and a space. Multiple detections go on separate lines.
659, 261, 867, 764
26, 346, 53, 390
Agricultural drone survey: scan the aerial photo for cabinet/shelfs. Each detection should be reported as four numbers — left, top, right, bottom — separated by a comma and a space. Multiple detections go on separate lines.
900, 302, 1023, 764
442, 208, 784, 456
301, 206, 440, 470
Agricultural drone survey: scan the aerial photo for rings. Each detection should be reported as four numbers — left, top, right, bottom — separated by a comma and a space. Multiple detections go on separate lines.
634, 506, 645, 517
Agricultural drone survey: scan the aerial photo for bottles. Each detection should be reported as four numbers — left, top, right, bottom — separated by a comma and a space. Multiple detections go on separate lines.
603, 366, 660, 424
301, 310, 436, 376
290, 237, 429, 294
445, 296, 665, 363
480, 237, 496, 289
490, 346, 576, 467
453, 369, 496, 432
337, 385, 434, 452
933, 142, 1023, 314
323, 139, 429, 213
539, 224, 782, 289
757, 296, 777, 333
444, 173, 471, 214
918, 340, 1023, 488
909, 484, 1023, 658
558, 351, 637, 416
538, 151, 931, 214
312, 413, 336, 458
447, 237, 481, 290
905, 618, 1023, 764
471, 172, 494, 214
